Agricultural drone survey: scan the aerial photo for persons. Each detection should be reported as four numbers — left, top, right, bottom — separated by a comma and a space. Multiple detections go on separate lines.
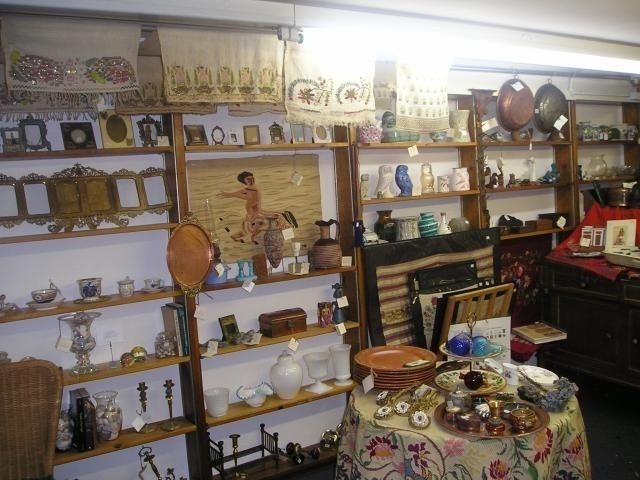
213, 171, 265, 245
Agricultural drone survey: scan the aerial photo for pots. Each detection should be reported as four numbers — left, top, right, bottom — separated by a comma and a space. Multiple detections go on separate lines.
498, 72, 568, 135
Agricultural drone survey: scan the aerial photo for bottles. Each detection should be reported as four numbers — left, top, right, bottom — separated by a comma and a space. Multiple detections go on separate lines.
437, 212, 453, 235
269, 350, 304, 401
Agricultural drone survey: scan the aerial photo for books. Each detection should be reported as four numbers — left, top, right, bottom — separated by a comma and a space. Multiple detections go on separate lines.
512, 321, 568, 344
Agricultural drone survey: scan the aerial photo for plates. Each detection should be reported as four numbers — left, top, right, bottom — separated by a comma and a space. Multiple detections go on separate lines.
141, 287, 165, 294
512, 362, 560, 388
351, 342, 437, 393
433, 395, 550, 441
25, 297, 67, 311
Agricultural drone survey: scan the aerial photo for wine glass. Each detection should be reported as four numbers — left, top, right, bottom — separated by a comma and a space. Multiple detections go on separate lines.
302, 343, 352, 395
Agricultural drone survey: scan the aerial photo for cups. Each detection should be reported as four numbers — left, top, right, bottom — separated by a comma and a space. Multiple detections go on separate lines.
77, 278, 104, 302
144, 278, 165, 289
31, 288, 58, 304
117, 277, 135, 299
202, 387, 230, 419
502, 363, 518, 386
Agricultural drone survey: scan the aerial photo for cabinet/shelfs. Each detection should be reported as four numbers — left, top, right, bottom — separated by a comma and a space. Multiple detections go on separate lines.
0, 92, 640, 479
